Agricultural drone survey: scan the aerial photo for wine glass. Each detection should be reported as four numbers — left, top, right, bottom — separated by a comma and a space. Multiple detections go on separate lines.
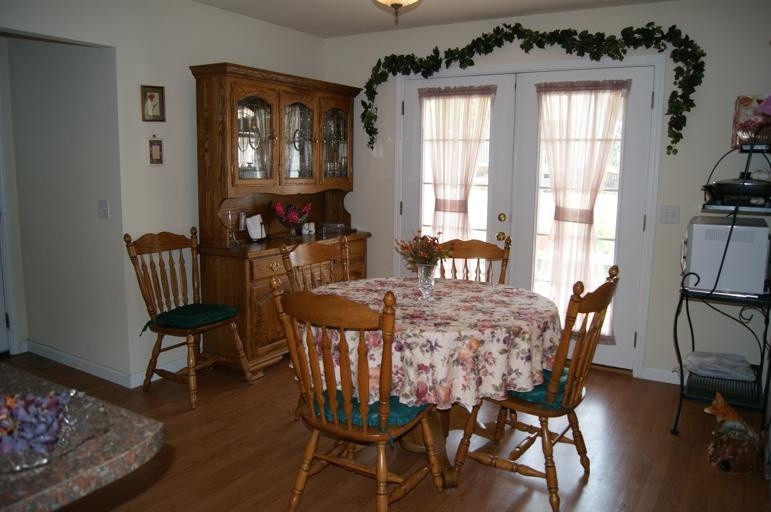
418, 264, 436, 302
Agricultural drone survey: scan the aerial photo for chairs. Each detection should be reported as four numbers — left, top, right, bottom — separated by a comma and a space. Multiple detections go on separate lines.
122, 225, 256, 410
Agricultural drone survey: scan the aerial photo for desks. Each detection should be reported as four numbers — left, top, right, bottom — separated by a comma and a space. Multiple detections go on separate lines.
0, 358, 165, 508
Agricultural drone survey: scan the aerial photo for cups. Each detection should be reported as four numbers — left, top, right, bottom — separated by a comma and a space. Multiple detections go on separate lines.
301, 222, 317, 235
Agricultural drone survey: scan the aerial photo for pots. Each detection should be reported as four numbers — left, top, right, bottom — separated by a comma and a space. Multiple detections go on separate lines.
704, 172, 771, 199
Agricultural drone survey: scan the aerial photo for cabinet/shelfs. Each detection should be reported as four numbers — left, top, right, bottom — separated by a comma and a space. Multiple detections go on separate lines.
668, 136, 770, 436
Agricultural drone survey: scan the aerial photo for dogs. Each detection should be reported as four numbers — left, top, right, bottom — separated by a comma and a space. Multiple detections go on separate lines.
703, 392, 752, 433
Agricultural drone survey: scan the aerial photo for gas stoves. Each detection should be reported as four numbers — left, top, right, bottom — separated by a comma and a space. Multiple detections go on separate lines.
704, 198, 771, 215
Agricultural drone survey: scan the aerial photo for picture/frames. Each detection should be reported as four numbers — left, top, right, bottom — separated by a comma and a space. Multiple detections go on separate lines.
139, 83, 166, 123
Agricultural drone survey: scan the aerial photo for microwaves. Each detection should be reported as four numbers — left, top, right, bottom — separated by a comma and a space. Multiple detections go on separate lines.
681, 216, 771, 295
